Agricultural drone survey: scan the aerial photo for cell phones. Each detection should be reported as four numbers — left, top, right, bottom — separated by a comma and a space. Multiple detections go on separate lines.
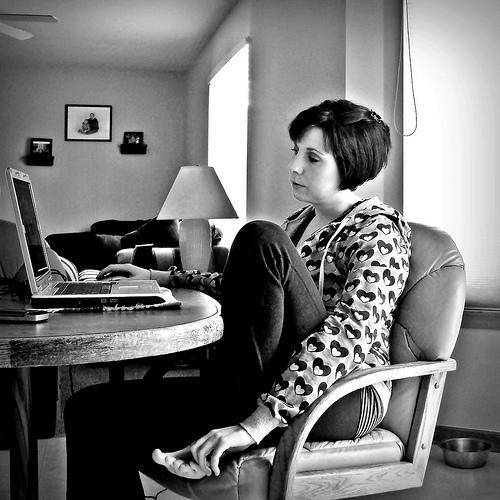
1, 309, 51, 324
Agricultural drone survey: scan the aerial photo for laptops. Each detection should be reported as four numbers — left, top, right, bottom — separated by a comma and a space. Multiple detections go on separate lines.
4, 166, 167, 308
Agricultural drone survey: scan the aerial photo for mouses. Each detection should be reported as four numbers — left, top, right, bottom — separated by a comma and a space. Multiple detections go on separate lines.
97, 273, 128, 281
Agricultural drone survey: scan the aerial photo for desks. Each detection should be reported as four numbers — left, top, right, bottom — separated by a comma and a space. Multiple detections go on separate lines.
0, 288, 223, 500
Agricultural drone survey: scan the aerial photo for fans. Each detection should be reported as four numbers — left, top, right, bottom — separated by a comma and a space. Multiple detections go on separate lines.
0, 13, 58, 40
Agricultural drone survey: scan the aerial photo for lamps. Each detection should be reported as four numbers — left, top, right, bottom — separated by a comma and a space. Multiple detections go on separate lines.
156, 165, 238, 273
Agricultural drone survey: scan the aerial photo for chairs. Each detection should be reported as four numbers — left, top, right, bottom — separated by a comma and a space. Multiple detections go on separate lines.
143, 222, 466, 500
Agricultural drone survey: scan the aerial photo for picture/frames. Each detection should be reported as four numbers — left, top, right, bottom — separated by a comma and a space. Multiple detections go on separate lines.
65, 104, 112, 142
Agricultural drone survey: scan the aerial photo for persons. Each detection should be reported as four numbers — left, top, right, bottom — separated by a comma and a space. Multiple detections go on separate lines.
61, 97, 414, 500
78, 111, 100, 134
81, 117, 91, 134
